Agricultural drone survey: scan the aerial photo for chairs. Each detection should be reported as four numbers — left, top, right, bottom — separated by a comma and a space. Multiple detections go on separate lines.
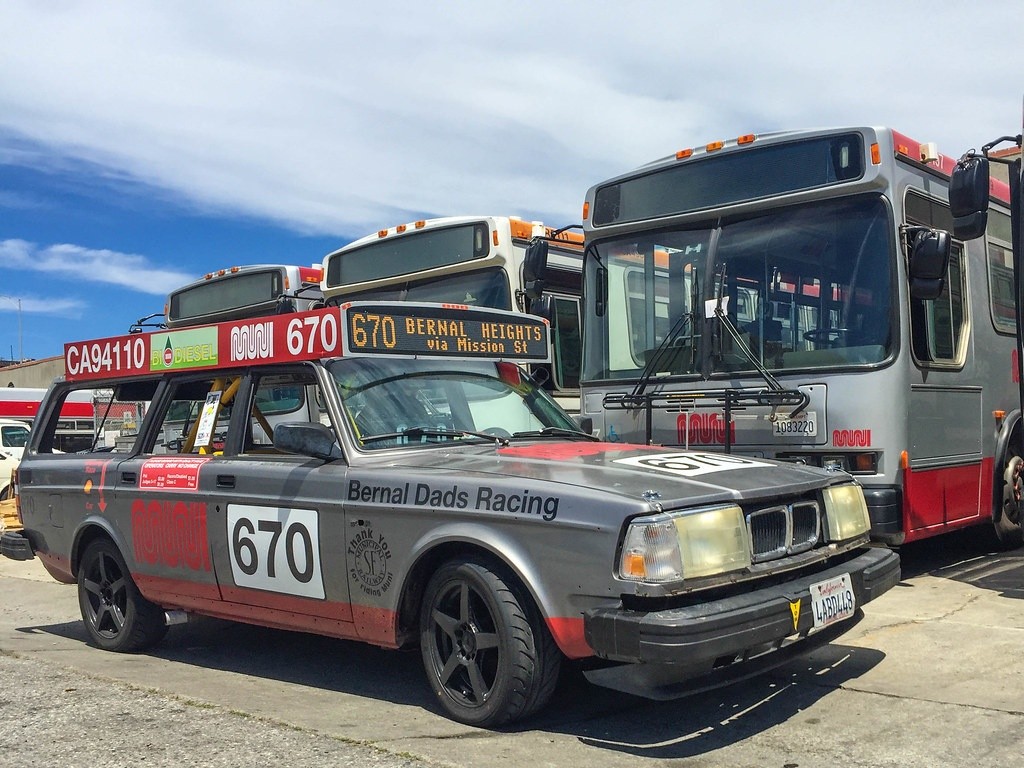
272, 422, 344, 461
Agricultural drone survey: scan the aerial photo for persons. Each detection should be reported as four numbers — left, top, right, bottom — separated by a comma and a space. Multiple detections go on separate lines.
355, 362, 431, 441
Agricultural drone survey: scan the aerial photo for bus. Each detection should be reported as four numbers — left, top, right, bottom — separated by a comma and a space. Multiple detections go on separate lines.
317, 216, 871, 441
524, 123, 1024, 553
0, 388, 164, 456
129, 260, 324, 335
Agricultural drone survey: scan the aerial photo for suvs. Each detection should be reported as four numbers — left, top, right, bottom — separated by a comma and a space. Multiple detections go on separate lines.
1, 298, 901, 730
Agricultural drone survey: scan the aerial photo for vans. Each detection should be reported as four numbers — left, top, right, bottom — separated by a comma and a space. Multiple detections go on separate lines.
0, 420, 67, 501
